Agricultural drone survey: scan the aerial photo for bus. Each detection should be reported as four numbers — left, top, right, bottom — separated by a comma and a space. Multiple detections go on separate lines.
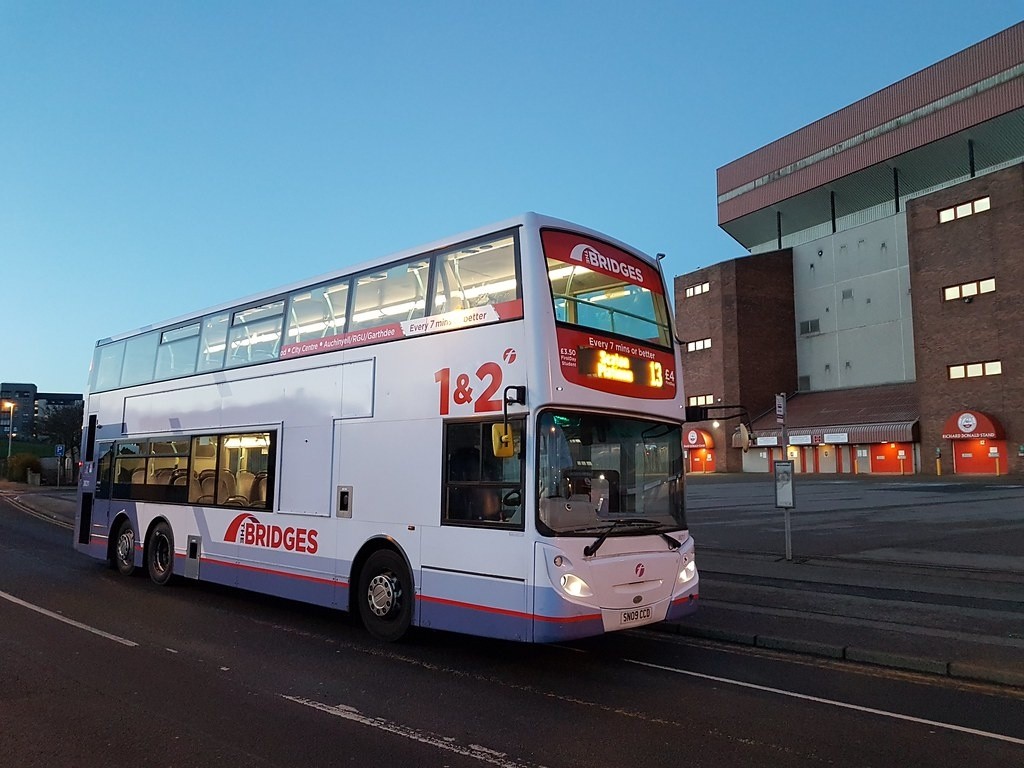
72, 211, 701, 645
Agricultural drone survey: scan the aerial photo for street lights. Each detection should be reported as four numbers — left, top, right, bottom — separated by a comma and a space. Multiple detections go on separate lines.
5, 402, 15, 458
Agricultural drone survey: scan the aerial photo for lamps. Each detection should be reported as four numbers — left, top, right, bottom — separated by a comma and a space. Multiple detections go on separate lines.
963, 297, 974, 303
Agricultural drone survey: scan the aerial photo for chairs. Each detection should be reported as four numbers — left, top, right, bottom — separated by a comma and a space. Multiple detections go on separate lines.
130, 466, 268, 509
